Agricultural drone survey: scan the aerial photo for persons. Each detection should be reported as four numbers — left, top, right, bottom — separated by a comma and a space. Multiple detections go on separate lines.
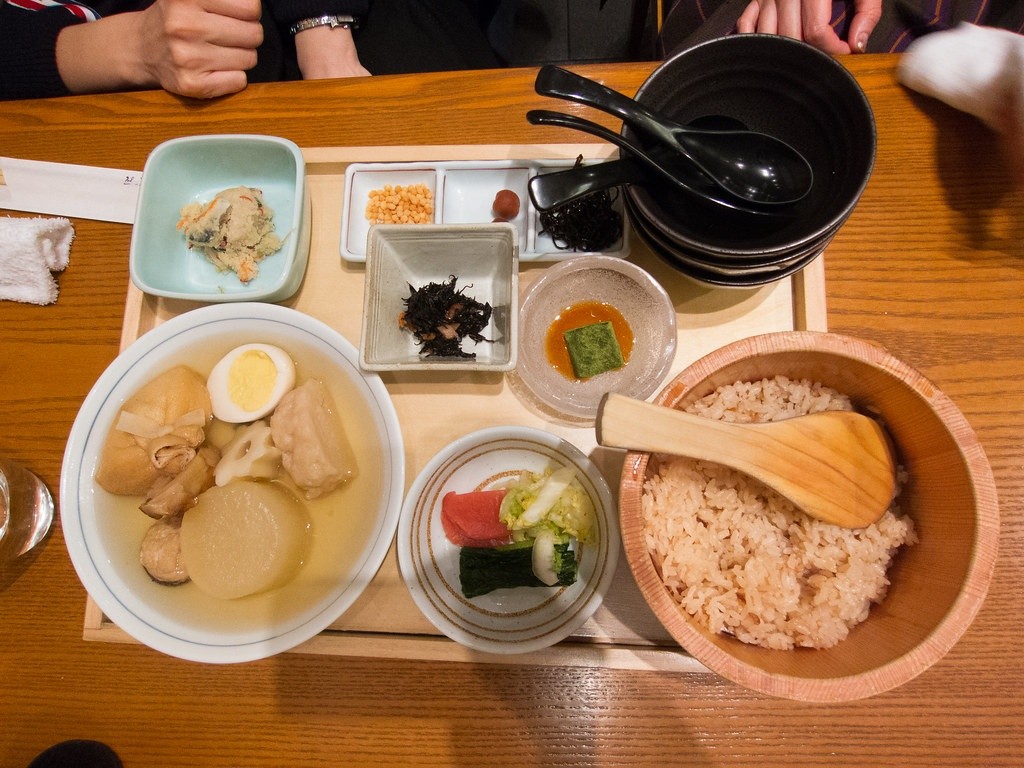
0, 0, 264, 101
662, 2, 1024, 75
260, 0, 662, 86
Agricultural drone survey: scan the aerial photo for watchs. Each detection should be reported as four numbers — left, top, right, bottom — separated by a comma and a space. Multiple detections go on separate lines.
288, 12, 361, 38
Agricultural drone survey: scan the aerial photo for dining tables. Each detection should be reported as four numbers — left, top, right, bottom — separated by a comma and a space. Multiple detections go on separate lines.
3, 51, 1015, 766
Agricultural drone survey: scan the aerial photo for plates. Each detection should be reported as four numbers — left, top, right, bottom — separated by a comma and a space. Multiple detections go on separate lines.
338, 160, 630, 261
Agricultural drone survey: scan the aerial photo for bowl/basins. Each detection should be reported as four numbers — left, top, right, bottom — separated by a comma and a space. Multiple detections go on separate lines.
358, 221, 520, 374
58, 303, 406, 666
129, 130, 314, 304
616, 327, 1000, 706
615, 34, 877, 259
625, 211, 836, 292
515, 254, 677, 421
392, 422, 620, 656
619, 187, 857, 278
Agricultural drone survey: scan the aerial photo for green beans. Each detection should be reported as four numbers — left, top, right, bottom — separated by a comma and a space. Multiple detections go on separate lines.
364, 180, 433, 227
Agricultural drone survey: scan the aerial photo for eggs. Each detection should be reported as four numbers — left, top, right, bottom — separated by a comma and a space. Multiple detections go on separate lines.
206, 341, 298, 427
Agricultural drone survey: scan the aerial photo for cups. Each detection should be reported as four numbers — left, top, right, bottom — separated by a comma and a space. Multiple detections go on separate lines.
0, 457, 55, 559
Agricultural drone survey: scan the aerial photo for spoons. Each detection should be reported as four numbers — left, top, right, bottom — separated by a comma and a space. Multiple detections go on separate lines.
532, 62, 817, 208
523, 107, 787, 220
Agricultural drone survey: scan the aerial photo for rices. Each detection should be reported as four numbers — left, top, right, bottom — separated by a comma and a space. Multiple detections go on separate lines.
641, 378, 922, 651
